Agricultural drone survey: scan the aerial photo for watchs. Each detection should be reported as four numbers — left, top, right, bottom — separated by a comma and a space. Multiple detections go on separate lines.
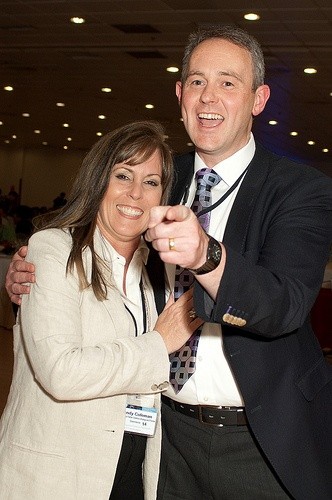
188, 233, 222, 275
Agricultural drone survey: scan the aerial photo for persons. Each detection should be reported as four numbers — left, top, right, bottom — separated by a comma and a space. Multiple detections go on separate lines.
0, 190, 68, 251
0, 121, 207, 500
6, 27, 332, 500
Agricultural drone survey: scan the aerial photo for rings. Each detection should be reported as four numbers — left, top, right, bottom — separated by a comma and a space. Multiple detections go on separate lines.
169, 238, 175, 250
190, 310, 196, 318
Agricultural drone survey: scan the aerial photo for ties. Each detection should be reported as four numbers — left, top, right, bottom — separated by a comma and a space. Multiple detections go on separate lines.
169, 169, 222, 395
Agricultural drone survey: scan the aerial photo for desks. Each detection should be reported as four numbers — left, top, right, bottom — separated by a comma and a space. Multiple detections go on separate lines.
0, 252, 15, 329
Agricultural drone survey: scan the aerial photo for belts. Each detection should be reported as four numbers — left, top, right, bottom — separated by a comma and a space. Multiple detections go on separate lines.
162, 394, 248, 427
